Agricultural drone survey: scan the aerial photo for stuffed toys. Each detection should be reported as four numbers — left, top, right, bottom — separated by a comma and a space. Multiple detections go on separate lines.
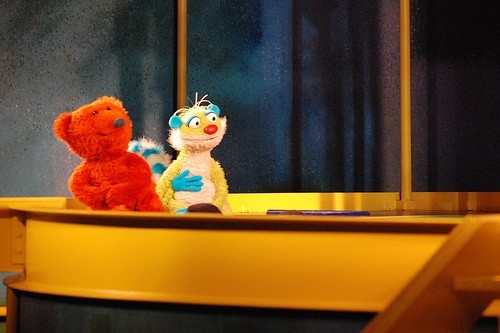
52, 95, 165, 211
127, 91, 230, 217
